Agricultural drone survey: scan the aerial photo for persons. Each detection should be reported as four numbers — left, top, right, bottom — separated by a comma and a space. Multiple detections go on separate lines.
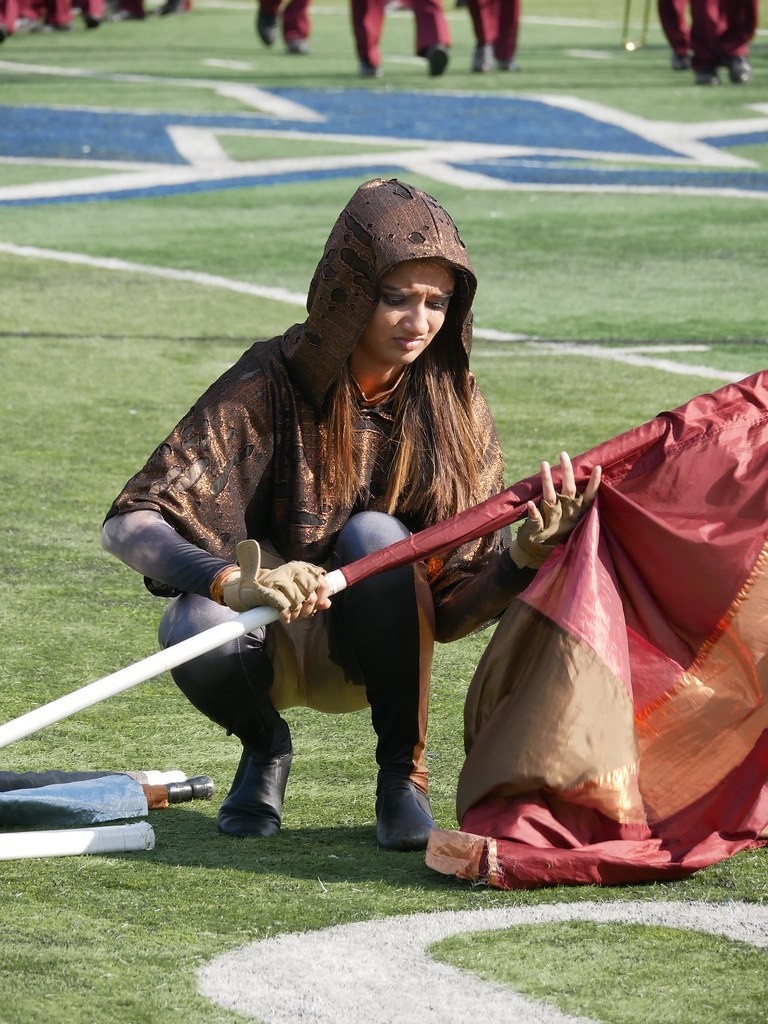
688, 0, 757, 85
257, 0, 314, 56
352, 0, 451, 77
658, 0, 695, 68
468, 0, 523, 72
99, 177, 604, 845
0, 0, 192, 44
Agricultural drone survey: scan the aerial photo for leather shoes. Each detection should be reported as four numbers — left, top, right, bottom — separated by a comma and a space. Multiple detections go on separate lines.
373, 767, 439, 850
215, 733, 295, 842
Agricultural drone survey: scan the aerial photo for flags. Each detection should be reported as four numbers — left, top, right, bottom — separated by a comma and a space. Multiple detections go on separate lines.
339, 368, 767, 884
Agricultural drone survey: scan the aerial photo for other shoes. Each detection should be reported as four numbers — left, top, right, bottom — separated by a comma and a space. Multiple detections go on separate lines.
288, 38, 310, 55
359, 64, 383, 78
254, 11, 279, 46
428, 45, 450, 76
502, 60, 520, 72
471, 44, 494, 73
671, 55, 693, 70
694, 72, 721, 86
728, 58, 753, 84
